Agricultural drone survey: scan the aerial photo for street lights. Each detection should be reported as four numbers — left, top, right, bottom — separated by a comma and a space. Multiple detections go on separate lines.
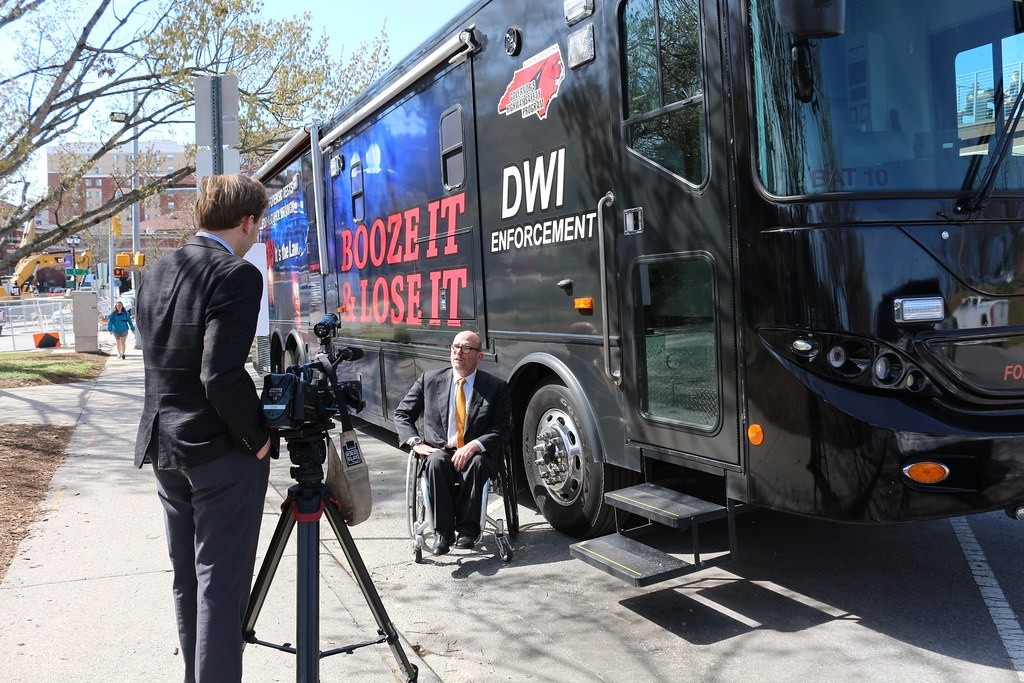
109, 111, 144, 351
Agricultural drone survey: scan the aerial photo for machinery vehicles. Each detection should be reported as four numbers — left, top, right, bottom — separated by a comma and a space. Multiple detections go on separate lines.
0, 214, 93, 299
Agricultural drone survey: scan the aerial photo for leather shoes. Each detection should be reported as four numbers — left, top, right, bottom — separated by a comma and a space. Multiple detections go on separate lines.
455, 525, 481, 549
431, 532, 456, 555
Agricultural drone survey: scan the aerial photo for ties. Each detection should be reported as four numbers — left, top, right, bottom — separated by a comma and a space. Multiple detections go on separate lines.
455, 378, 466, 449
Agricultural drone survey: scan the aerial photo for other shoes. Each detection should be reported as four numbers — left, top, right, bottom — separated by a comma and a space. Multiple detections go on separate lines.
117, 354, 121, 358
122, 355, 126, 360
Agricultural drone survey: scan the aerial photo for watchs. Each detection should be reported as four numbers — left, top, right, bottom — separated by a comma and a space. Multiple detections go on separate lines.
411, 437, 423, 449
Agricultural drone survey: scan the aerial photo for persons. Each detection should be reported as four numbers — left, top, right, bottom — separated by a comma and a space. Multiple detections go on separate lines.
566, 322, 631, 441
133, 174, 272, 683
393, 330, 510, 556
108, 301, 135, 359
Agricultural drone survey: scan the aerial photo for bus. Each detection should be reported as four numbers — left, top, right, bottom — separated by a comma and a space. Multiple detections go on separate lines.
245, 0, 1024, 588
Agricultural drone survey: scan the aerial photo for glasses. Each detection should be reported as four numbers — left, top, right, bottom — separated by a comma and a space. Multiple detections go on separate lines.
450, 345, 481, 354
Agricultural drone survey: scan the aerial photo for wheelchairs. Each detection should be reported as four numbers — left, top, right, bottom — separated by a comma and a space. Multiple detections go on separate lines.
404, 426, 519, 564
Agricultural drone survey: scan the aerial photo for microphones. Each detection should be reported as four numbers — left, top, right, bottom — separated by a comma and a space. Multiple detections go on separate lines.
341, 346, 364, 361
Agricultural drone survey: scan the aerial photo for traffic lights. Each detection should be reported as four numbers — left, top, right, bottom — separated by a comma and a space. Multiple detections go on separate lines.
111, 216, 122, 237
114, 268, 125, 277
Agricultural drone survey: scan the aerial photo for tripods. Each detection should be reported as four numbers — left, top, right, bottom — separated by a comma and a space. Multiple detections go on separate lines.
240, 425, 419, 683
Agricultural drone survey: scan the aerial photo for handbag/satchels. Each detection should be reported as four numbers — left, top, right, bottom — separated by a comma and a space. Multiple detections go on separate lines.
324, 352, 372, 526
